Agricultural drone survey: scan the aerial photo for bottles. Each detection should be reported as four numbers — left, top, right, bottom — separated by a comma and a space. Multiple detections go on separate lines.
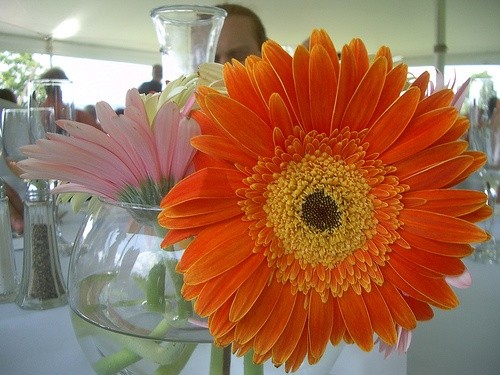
149, 5, 228, 92
0, 185, 20, 304
15, 179, 69, 310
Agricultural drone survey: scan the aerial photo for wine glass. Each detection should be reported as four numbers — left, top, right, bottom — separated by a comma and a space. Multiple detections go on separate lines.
463, 78, 500, 264
0, 107, 72, 252
27, 80, 74, 258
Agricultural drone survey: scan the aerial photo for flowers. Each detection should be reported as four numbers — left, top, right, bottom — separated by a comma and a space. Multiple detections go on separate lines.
12, 29, 493, 374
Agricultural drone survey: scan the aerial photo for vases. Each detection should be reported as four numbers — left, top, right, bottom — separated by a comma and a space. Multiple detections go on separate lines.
0, 4, 233, 375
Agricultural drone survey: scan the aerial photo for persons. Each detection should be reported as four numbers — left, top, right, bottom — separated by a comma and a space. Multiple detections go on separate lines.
485, 96, 500, 166
0, 64, 163, 231
191, 4, 266, 68
